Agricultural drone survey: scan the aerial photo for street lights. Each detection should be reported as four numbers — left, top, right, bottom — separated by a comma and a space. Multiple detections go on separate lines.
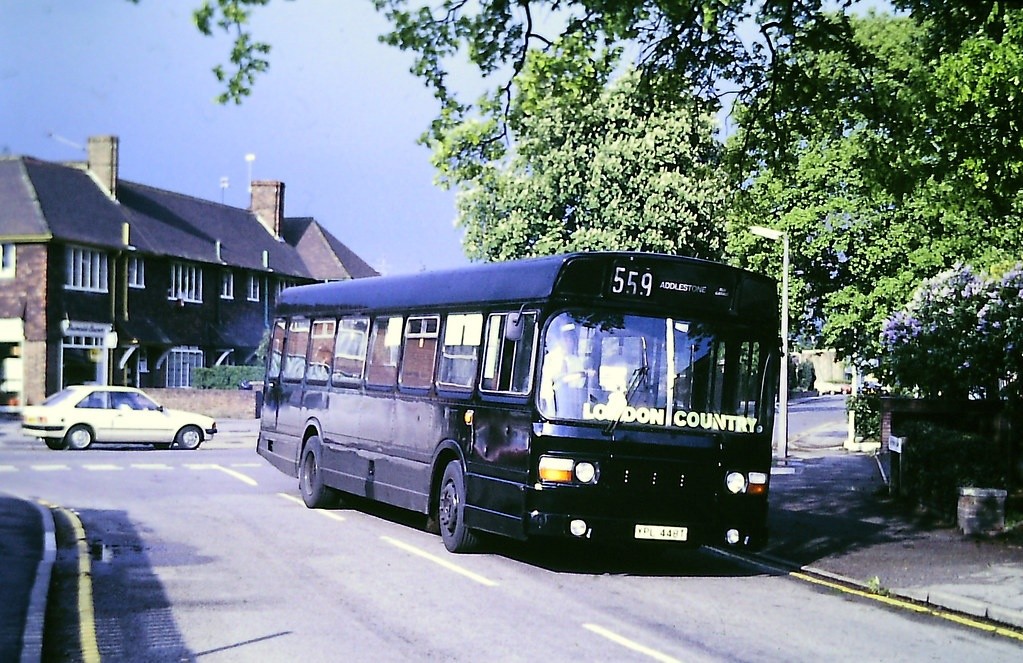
748, 225, 795, 475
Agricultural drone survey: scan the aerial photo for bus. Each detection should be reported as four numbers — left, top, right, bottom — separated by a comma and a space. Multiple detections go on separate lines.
255, 252, 785, 554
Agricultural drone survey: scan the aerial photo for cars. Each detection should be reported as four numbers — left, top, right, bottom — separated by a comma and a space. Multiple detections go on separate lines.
814, 382, 852, 397
21, 385, 217, 450
